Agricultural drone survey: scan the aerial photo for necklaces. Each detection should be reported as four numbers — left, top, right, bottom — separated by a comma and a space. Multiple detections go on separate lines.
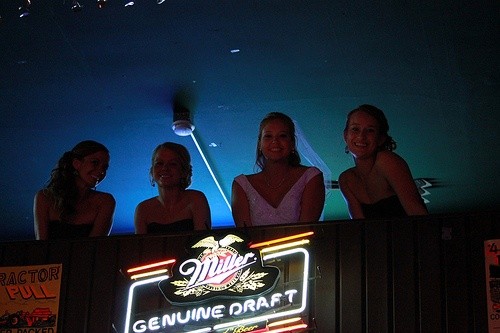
264, 167, 287, 194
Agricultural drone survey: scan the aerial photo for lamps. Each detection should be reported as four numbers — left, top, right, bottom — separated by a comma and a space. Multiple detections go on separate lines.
172, 121, 195, 136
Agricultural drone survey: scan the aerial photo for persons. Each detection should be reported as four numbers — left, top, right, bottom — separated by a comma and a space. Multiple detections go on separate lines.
231, 113, 325, 227
34, 140, 116, 239
134, 142, 211, 235
338, 104, 429, 219
